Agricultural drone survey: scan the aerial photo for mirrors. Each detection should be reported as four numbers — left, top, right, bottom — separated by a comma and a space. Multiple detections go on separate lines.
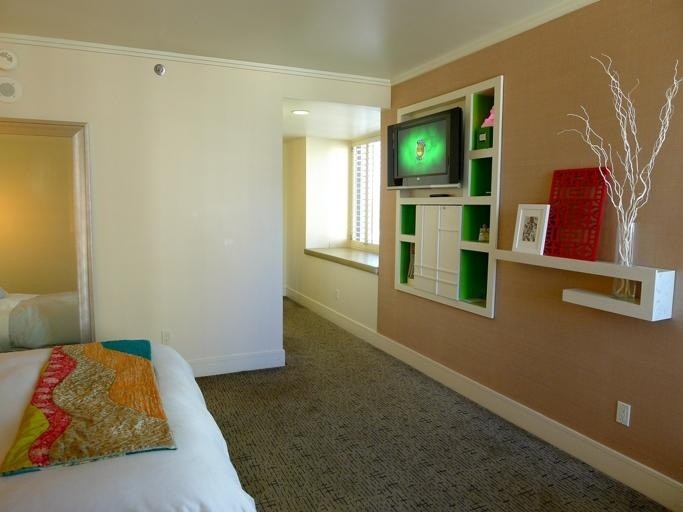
0, 118, 92, 354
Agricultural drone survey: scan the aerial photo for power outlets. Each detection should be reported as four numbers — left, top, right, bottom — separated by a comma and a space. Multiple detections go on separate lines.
616, 400, 631, 427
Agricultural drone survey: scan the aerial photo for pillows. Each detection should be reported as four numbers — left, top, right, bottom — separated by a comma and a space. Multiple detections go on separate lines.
0, 285, 10, 299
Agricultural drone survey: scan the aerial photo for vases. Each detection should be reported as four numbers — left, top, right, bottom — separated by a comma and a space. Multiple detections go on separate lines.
614, 221, 638, 298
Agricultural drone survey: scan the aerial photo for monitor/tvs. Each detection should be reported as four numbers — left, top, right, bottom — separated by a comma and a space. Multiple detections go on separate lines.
386, 107, 464, 187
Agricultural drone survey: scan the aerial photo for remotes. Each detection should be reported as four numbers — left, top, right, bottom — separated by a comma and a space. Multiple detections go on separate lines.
429, 193, 450, 197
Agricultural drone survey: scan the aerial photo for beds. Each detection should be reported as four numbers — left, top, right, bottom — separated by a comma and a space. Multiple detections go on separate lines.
0, 293, 79, 349
0, 337, 255, 512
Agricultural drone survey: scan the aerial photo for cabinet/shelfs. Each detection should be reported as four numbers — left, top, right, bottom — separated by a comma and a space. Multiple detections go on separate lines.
393, 74, 504, 319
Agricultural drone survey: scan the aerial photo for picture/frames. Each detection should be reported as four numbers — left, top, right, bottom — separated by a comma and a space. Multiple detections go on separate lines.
512, 203, 551, 255
